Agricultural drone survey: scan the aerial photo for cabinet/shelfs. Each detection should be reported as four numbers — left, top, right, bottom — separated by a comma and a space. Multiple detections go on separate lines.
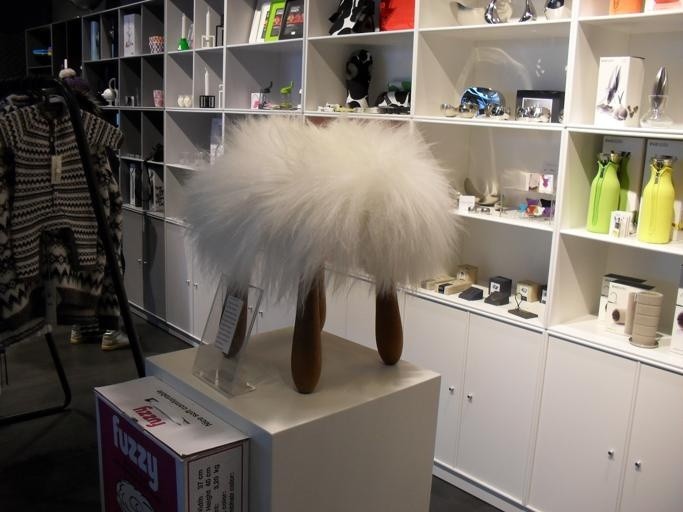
24, 2, 166, 325
417, 3, 682, 509
168, 0, 415, 363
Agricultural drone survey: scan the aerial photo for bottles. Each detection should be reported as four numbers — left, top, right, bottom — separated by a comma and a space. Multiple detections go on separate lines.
279, 80, 295, 109
586, 152, 622, 234
177, 39, 188, 51
262, 81, 273, 108
636, 153, 674, 244
640, 95, 675, 128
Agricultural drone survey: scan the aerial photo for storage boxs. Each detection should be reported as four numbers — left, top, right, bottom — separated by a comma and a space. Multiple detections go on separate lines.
94, 375, 253, 510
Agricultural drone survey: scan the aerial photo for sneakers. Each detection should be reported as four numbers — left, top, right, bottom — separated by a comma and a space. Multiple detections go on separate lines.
101, 329, 141, 351
70, 324, 89, 344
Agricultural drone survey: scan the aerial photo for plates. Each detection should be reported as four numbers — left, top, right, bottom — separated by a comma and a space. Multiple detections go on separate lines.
460, 88, 501, 117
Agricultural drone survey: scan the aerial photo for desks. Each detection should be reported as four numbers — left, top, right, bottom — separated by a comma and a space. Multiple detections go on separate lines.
146, 324, 442, 512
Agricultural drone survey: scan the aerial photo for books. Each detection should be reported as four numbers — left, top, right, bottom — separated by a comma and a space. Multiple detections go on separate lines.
248, 0, 304, 46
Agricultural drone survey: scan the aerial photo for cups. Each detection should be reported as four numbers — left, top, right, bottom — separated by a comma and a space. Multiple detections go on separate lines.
153, 89, 164, 107
250, 92, 264, 108
148, 35, 164, 53
627, 291, 664, 346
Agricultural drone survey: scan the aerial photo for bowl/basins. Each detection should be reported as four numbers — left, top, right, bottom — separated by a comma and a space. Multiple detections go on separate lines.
438, 103, 564, 123
